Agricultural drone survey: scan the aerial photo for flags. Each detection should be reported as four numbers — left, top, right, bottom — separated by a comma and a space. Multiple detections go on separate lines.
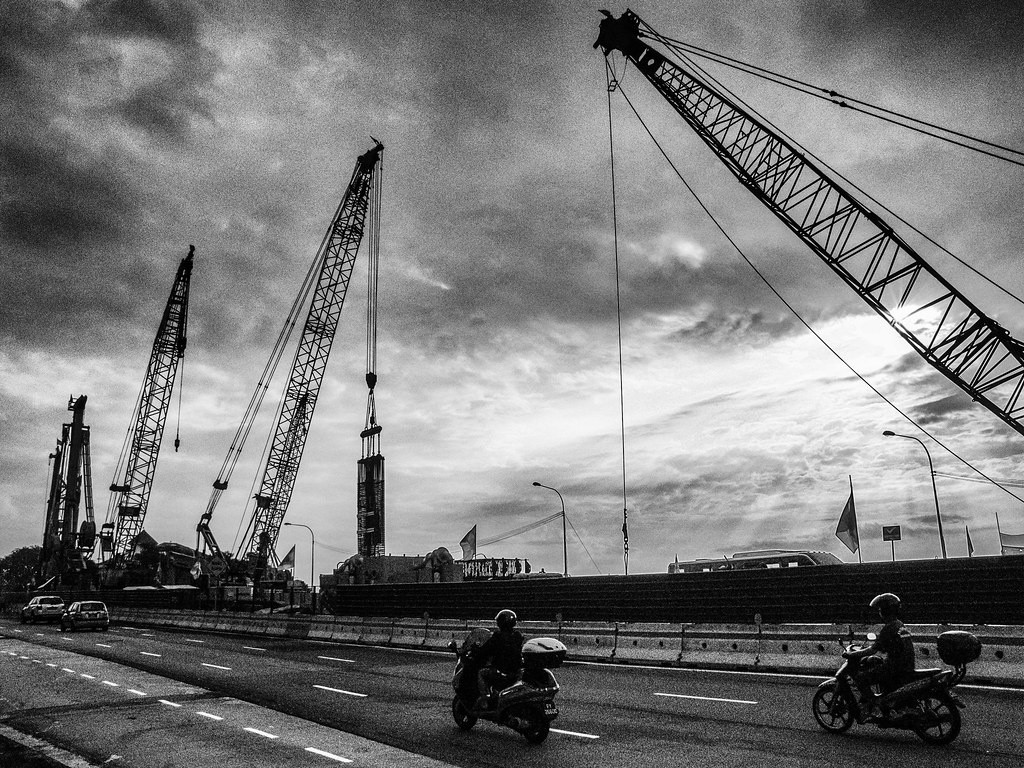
460, 525, 476, 561
835, 478, 860, 554
190, 557, 202, 578
966, 527, 974, 554
277, 546, 295, 570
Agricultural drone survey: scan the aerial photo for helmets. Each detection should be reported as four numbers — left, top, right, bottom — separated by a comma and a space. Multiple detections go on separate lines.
494, 609, 518, 632
870, 593, 901, 622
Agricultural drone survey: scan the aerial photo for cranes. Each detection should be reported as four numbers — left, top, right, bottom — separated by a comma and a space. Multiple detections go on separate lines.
95, 242, 196, 597
191, 131, 389, 604
32, 392, 96, 591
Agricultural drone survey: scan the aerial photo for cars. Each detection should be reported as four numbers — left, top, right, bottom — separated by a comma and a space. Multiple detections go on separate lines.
60, 599, 110, 633
20, 595, 66, 625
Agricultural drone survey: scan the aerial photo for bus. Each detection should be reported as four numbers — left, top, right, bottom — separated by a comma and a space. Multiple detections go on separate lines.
668, 549, 845, 574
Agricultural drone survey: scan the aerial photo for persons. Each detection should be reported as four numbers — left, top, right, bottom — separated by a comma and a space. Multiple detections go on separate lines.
842, 593, 915, 724
461, 610, 524, 710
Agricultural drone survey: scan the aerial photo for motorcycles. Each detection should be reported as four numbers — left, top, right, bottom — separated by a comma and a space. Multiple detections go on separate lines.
811, 627, 980, 747
443, 631, 568, 745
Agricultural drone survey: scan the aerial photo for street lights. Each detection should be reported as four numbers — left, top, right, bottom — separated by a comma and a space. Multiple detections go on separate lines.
597, 6, 1024, 511
881, 427, 950, 560
284, 519, 316, 587
532, 480, 572, 577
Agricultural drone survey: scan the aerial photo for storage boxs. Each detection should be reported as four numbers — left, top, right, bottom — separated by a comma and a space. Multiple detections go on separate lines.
521, 638, 568, 666
937, 630, 982, 662
